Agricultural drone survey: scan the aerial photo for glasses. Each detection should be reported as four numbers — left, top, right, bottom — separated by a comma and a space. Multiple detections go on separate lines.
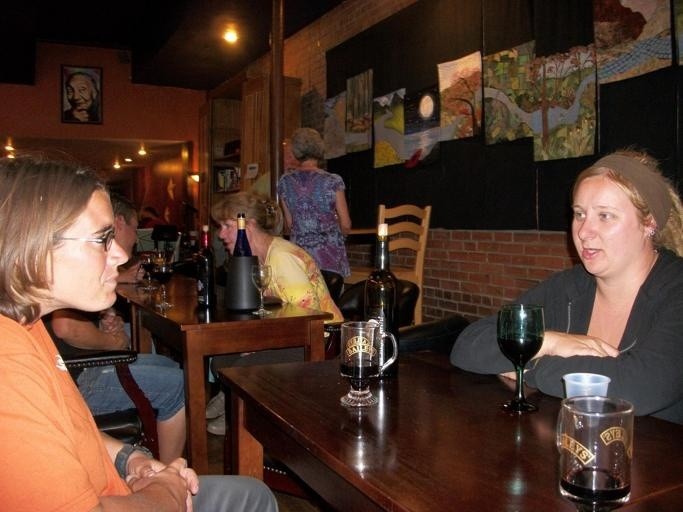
53, 225, 116, 252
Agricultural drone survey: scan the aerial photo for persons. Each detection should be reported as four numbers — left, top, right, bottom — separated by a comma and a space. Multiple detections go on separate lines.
211, 191, 344, 350
0, 146, 279, 512
275, 126, 353, 304
39, 186, 188, 466
61, 71, 102, 123
448, 149, 683, 424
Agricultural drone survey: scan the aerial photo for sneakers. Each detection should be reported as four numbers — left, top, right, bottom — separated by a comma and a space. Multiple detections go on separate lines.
205, 390, 225, 418
207, 414, 226, 436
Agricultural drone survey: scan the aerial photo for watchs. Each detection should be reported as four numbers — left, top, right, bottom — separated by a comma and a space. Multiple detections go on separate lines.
114, 444, 154, 480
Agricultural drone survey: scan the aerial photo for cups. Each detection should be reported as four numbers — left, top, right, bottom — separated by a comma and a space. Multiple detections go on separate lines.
555, 394, 632, 511
561, 370, 611, 428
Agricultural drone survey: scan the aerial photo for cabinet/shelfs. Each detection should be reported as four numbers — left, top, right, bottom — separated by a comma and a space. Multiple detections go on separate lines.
198, 70, 301, 225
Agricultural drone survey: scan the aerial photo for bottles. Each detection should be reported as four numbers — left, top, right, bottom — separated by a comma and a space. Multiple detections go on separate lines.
232, 211, 252, 257
194, 306, 217, 324
194, 223, 218, 307
364, 222, 399, 378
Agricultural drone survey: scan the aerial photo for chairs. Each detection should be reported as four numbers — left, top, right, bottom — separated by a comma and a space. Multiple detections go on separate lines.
64, 351, 159, 463
324, 279, 419, 360
344, 204, 432, 331
321, 269, 344, 303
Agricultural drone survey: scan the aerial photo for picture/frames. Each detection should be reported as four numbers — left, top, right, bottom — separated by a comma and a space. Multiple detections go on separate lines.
60, 63, 103, 124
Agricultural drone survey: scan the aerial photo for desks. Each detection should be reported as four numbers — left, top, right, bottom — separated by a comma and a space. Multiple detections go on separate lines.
129, 293, 333, 476
216, 351, 683, 512
116, 268, 200, 351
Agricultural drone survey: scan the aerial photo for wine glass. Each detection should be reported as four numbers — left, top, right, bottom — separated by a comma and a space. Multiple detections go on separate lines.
495, 303, 552, 414
138, 245, 177, 313
250, 264, 273, 317
337, 320, 384, 407
